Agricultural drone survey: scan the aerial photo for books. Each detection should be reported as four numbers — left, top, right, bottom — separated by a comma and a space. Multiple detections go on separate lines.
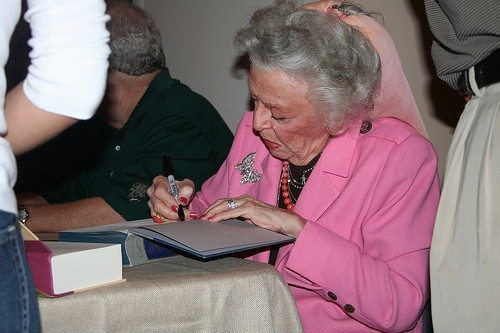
60, 218, 298, 268
18, 220, 127, 298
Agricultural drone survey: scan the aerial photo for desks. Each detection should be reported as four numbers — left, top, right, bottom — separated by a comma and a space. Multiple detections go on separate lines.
36, 232, 304, 333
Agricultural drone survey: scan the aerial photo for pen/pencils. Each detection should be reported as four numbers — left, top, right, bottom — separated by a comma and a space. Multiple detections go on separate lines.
163, 153, 186, 222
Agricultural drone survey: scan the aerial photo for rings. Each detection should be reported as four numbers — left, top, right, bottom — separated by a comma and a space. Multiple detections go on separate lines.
153, 214, 167, 223
227, 199, 237, 210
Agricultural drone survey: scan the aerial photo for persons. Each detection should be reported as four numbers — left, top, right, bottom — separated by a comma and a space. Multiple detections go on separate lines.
0, 0, 110, 333
424, 0, 500, 333
17, 0, 234, 233
146, 0, 440, 333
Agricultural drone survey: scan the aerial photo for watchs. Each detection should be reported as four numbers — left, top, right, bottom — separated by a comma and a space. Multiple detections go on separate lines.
18, 206, 29, 225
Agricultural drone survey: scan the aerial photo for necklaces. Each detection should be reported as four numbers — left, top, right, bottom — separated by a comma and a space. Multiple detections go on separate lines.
281, 160, 314, 208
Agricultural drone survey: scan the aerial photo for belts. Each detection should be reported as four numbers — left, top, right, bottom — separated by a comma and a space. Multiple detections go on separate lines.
457, 47, 500, 96
